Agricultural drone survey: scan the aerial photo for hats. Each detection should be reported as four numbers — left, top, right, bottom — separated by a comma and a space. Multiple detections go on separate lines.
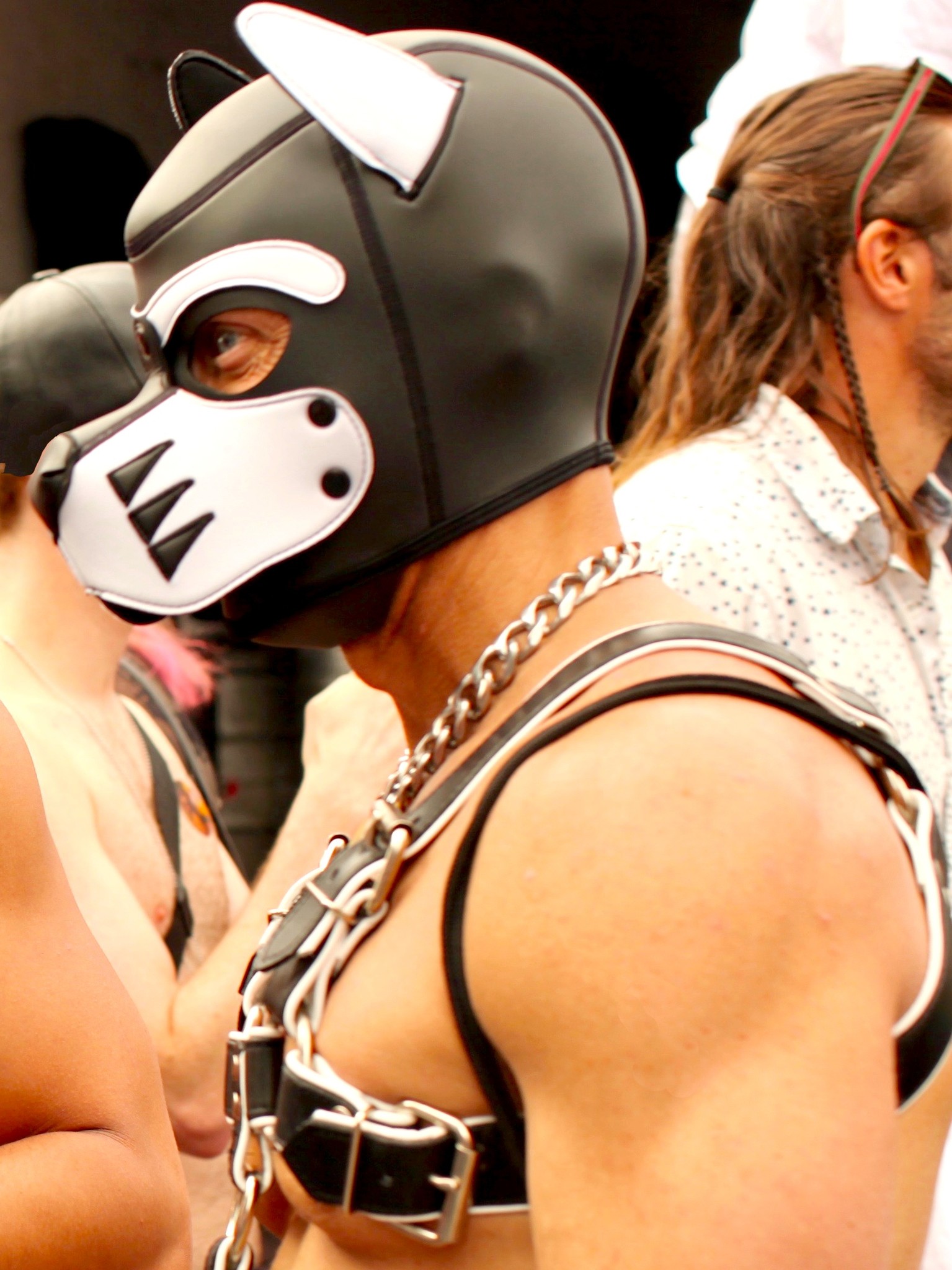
0, 264, 176, 472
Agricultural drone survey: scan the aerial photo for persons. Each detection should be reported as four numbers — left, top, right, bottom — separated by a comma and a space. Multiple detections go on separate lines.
614, 57, 951, 819
5, 257, 411, 1267
2, 710, 194, 1264
24, 1, 951, 1270
